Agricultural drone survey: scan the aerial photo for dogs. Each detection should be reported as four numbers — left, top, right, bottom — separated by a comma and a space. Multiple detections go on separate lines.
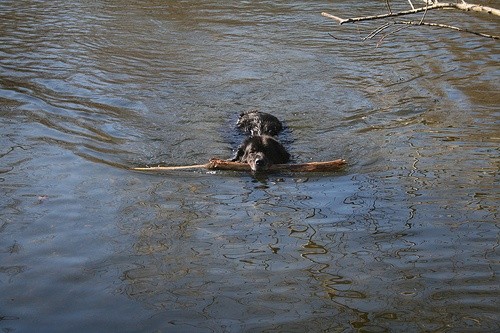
220, 109, 291, 172
